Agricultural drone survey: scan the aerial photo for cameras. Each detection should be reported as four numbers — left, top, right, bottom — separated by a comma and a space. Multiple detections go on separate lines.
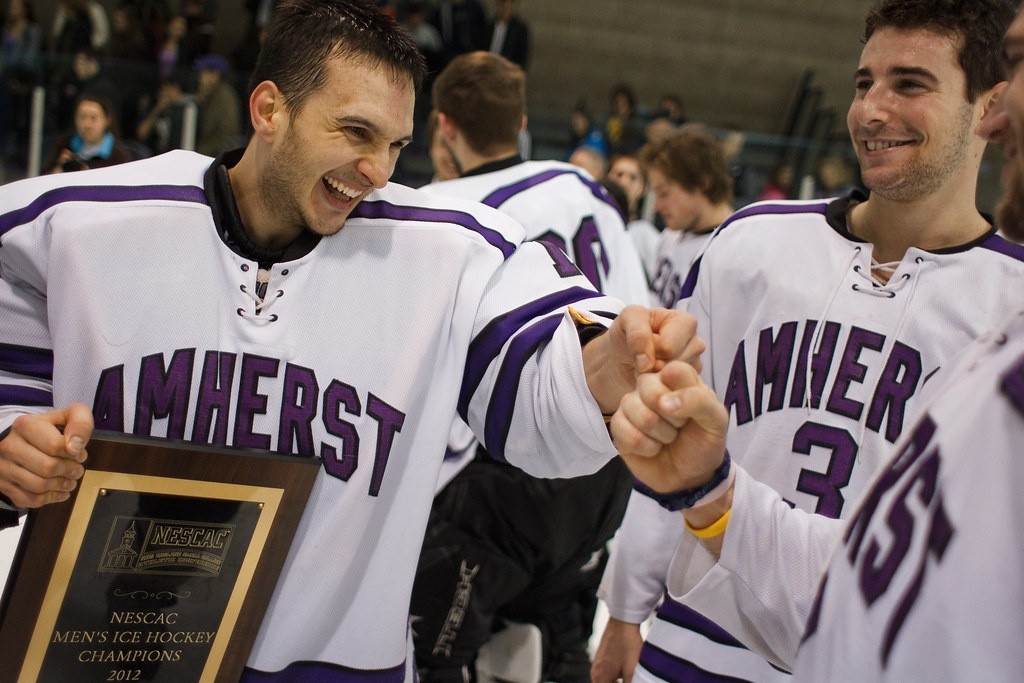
62, 158, 83, 172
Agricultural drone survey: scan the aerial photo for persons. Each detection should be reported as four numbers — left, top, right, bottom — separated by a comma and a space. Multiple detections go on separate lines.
405, 50, 651, 683
0, 0, 1024, 683
609, 8, 1024, 683
589, 2, 1008, 681
1, 0, 707, 681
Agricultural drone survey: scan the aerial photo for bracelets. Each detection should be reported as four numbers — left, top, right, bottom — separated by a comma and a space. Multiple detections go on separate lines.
681, 460, 736, 509
683, 511, 732, 539
658, 449, 732, 512
